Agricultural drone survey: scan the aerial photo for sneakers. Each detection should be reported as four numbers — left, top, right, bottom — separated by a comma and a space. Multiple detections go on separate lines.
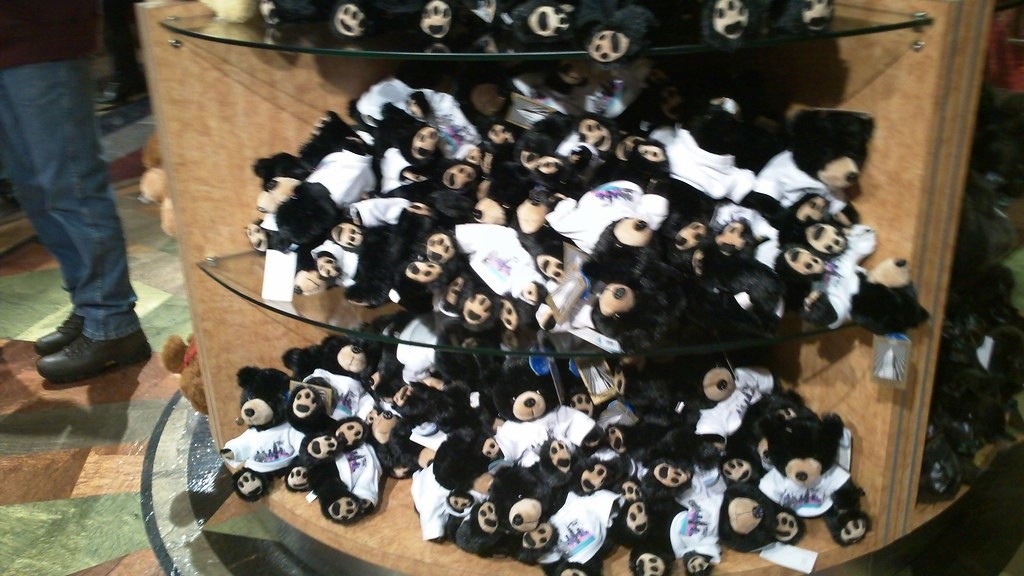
36, 327, 151, 384
33, 313, 85, 356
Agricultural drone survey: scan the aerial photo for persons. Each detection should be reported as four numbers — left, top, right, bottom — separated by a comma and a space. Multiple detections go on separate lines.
0, 0, 152, 383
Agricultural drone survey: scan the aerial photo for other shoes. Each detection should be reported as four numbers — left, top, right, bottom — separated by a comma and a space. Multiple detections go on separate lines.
94, 71, 145, 104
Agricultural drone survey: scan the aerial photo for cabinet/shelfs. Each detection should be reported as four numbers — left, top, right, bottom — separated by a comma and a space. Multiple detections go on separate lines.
133, 0, 1024, 576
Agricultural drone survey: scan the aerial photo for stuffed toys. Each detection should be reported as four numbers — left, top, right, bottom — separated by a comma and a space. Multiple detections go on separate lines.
139, 1, 1024, 576
